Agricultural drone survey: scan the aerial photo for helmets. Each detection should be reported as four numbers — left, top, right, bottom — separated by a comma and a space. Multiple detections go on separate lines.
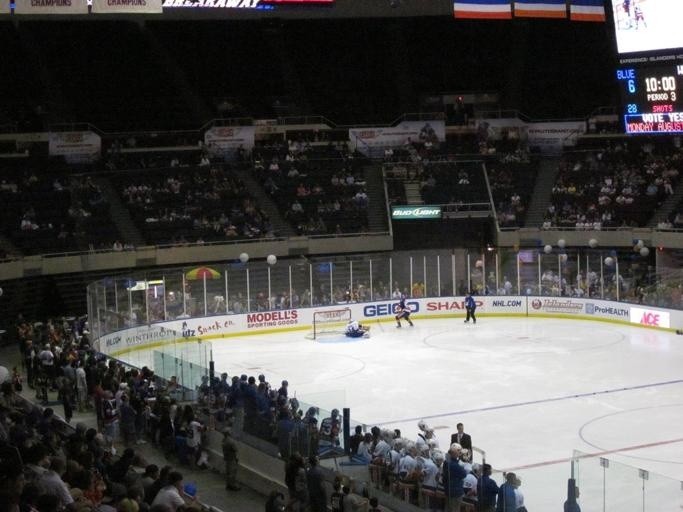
381, 420, 481, 475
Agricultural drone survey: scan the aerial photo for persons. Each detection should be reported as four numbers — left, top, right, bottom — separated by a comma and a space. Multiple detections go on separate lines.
105, 136, 277, 246
1, 174, 133, 253
252, 130, 369, 234
386, 120, 531, 229
463, 293, 477, 324
460, 258, 532, 295
562, 486, 582, 512
105, 276, 424, 324
318, 409, 528, 512
1, 314, 327, 512
395, 296, 413, 328
532, 114, 682, 311
344, 319, 370, 337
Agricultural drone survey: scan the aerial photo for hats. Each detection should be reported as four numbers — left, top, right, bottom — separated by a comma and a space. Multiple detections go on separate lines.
118, 498, 139, 512
83, 330, 90, 335
120, 383, 128, 389
